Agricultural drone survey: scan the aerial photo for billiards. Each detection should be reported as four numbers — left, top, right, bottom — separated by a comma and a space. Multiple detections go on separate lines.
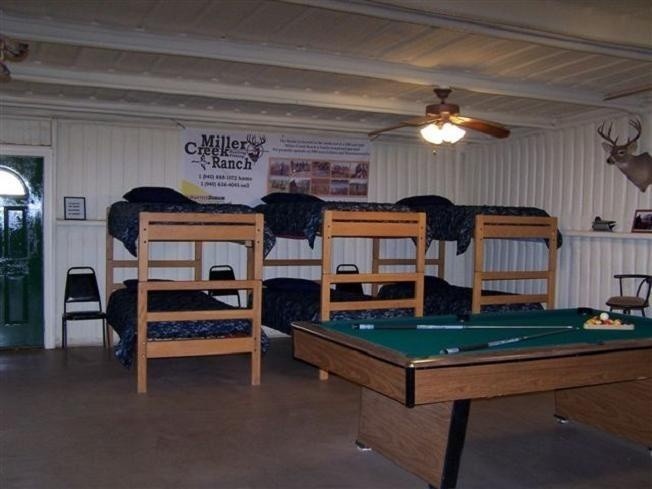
586, 313, 630, 325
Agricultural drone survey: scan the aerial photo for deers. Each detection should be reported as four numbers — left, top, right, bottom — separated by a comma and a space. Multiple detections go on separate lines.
596, 117, 652, 193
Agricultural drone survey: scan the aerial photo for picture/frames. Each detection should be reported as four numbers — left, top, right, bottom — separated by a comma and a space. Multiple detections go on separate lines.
65, 196, 86, 220
631, 210, 652, 233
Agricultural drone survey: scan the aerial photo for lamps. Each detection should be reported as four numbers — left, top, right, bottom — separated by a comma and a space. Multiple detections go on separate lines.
421, 121, 466, 145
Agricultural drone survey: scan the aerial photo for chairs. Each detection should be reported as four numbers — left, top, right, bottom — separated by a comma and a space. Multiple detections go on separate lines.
64, 264, 108, 351
607, 274, 651, 318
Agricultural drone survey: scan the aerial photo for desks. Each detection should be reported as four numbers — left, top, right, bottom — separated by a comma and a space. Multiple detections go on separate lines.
290, 307, 651, 489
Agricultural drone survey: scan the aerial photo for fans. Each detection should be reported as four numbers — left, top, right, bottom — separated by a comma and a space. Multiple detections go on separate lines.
368, 89, 511, 160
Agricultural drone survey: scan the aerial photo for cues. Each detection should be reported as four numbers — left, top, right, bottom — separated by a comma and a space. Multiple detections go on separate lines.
352, 323, 573, 339
440, 327, 580, 354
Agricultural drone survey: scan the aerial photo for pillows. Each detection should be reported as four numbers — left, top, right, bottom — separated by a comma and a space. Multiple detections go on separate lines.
396, 195, 453, 206
122, 186, 195, 203
261, 192, 325, 203
